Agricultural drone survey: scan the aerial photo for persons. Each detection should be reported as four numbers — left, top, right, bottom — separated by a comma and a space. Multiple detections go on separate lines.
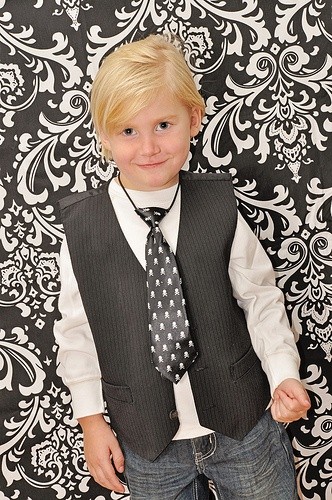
54, 33, 310, 500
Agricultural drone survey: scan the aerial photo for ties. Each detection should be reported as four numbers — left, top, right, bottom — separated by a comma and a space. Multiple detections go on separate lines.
135, 207, 198, 385
